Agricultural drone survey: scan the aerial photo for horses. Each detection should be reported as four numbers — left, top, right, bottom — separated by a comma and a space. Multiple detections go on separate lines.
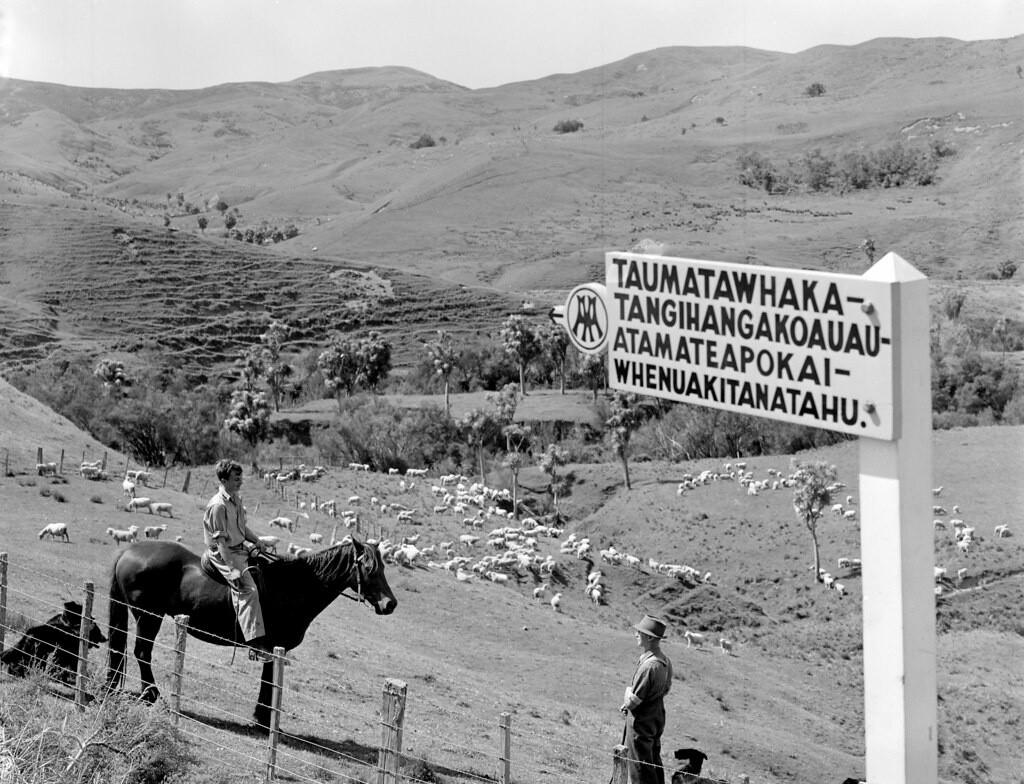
106, 535, 398, 732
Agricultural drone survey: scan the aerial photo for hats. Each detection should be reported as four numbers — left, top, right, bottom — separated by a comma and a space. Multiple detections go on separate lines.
633, 615, 667, 639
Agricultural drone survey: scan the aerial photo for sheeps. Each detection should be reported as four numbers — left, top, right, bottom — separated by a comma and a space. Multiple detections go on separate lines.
678, 463, 1013, 595
36, 460, 735, 655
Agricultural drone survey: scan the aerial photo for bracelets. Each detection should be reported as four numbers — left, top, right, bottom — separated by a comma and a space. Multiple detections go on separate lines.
230, 566, 236, 572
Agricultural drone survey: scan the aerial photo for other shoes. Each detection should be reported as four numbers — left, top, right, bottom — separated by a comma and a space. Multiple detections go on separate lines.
248, 648, 275, 663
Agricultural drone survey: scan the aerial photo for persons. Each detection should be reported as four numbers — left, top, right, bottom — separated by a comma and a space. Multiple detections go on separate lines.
201, 459, 272, 660
619, 615, 672, 784
